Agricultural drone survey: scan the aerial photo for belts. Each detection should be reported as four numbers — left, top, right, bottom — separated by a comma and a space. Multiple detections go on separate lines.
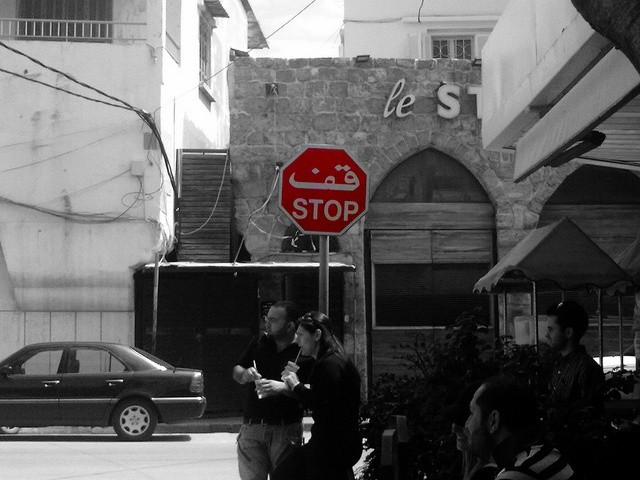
242, 415, 302, 425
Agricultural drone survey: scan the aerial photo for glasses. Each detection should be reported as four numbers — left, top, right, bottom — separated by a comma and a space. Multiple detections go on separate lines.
265, 315, 288, 323
294, 333, 315, 338
554, 300, 567, 310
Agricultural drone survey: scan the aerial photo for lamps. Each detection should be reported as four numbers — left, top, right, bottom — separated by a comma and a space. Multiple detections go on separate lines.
472, 59, 481, 66
357, 55, 369, 63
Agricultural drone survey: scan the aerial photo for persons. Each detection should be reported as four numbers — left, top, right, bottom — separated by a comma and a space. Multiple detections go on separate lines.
232, 299, 315, 480
465, 378, 578, 479
545, 300, 606, 479
442, 366, 492, 479
270, 310, 363, 480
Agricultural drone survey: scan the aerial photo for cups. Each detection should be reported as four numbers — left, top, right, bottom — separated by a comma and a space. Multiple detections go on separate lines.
286, 362, 300, 382
254, 377, 266, 399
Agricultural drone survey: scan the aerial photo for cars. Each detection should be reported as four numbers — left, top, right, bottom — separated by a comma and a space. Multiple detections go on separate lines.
0, 342, 206, 441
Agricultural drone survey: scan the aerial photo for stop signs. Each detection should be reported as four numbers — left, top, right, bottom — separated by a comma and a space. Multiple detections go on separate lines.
278, 147, 368, 236
